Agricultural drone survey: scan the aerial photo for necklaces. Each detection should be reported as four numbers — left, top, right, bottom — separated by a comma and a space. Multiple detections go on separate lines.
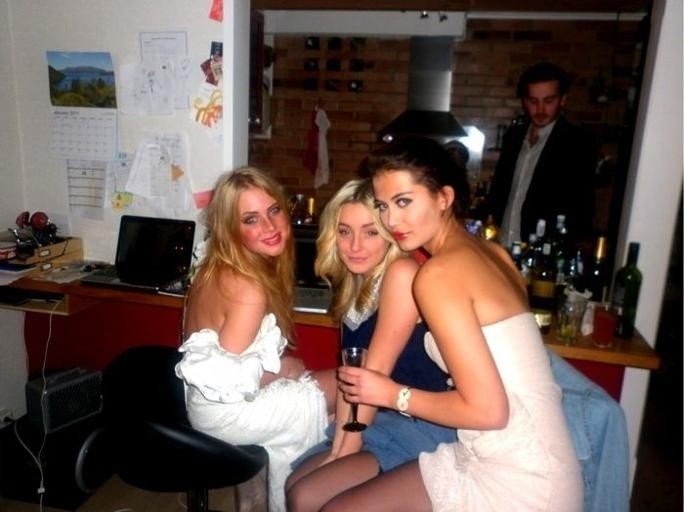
346, 269, 381, 323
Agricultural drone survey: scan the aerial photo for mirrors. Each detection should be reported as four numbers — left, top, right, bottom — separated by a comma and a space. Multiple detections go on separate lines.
251, 4, 650, 311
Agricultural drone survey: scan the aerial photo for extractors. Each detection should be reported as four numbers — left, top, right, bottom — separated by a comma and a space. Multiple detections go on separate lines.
375, 35, 471, 139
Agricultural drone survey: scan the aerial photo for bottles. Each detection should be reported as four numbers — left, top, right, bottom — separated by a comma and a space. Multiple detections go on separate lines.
496, 119, 519, 150
585, 234, 610, 303
305, 195, 316, 224
506, 213, 585, 336
609, 241, 643, 340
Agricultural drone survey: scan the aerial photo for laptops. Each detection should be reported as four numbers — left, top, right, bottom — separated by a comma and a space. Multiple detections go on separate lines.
292, 238, 334, 315
79, 214, 196, 292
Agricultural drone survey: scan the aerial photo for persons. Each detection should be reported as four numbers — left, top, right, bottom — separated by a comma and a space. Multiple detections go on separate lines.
318, 134, 586, 512
175, 165, 337, 512
468, 63, 597, 254
284, 178, 457, 512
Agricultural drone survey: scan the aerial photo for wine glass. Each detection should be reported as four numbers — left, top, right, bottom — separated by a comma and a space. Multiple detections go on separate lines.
340, 347, 369, 432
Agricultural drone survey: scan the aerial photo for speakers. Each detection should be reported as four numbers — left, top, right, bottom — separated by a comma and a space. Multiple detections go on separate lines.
25, 368, 103, 435
0, 410, 116, 512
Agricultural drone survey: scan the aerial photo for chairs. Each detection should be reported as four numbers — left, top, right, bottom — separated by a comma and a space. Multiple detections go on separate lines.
101, 344, 270, 512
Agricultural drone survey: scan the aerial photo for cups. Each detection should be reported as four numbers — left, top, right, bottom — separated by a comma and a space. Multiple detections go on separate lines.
0, 241, 17, 261
591, 301, 620, 349
553, 293, 587, 345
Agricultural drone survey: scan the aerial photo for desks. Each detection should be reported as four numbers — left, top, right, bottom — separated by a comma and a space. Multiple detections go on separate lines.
19, 275, 661, 404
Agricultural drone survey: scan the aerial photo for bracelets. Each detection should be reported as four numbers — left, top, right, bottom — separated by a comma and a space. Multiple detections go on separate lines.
396, 387, 411, 418
